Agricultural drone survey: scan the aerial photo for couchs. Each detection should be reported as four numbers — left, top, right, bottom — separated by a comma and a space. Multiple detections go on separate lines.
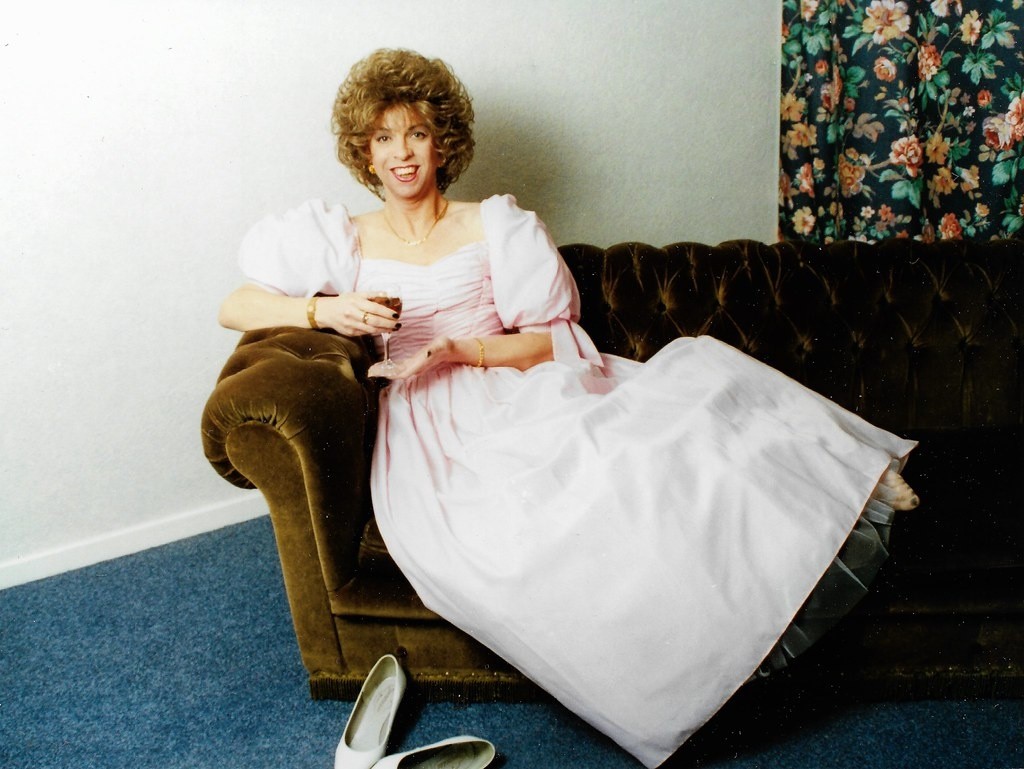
201, 238, 1024, 700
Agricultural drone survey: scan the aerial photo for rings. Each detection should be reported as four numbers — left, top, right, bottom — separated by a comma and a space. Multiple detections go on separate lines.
363, 312, 368, 324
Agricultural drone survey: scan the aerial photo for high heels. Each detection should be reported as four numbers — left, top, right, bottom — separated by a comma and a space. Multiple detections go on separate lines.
331, 652, 406, 769
371, 735, 496, 769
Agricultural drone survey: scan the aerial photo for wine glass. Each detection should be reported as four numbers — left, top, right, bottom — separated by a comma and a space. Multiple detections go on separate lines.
365, 281, 402, 376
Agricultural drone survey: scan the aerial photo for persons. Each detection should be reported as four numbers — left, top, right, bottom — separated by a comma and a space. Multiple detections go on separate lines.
215, 49, 920, 769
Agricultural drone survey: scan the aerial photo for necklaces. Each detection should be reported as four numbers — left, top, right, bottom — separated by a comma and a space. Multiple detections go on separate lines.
380, 200, 449, 245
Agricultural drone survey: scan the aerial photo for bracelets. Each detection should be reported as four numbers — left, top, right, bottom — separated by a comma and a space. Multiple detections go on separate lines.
471, 338, 484, 367
307, 296, 319, 329
